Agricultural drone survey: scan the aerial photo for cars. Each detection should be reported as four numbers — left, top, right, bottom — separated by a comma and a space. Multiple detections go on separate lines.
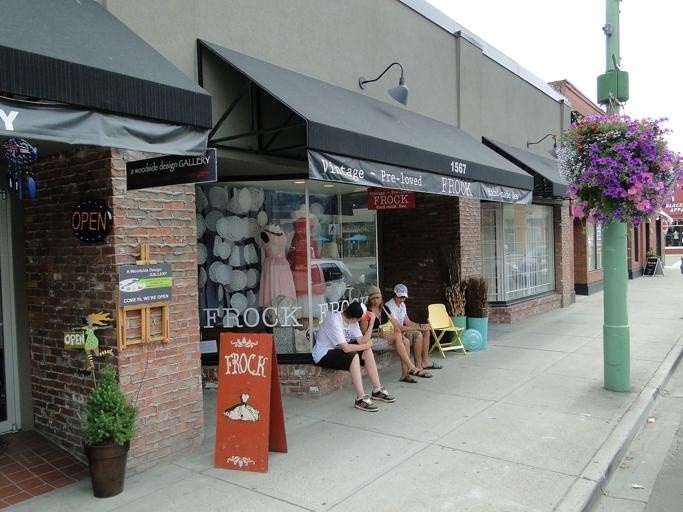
511, 245, 550, 284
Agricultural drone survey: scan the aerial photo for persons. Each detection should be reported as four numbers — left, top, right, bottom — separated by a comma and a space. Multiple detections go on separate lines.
385, 284, 443, 371
257, 203, 327, 307
359, 284, 433, 384
310, 300, 396, 413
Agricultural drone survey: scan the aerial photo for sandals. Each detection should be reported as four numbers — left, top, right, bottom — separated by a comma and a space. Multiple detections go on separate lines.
399, 375, 418, 383
408, 367, 425, 376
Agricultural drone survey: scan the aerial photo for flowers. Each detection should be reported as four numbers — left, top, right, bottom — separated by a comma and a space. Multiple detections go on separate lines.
556, 115, 683, 226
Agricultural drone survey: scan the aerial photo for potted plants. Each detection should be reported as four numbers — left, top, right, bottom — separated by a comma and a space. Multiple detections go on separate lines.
80, 361, 140, 497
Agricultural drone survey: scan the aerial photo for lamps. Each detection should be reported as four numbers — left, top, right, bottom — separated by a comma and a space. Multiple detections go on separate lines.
526, 133, 558, 160
358, 61, 408, 107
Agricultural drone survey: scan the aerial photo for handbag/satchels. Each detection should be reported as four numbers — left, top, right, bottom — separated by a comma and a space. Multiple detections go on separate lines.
378, 319, 394, 334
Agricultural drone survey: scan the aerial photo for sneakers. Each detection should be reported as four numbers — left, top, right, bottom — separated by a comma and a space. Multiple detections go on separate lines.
354, 394, 380, 412
371, 386, 396, 403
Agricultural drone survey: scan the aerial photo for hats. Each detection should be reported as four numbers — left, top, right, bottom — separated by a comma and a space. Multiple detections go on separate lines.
367, 285, 381, 300
393, 284, 409, 299
348, 302, 371, 321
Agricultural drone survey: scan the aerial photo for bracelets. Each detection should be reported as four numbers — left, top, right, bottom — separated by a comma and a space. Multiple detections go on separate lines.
419, 324, 421, 328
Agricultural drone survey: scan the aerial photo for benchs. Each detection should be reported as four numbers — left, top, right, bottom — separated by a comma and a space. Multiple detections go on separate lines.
199, 353, 319, 397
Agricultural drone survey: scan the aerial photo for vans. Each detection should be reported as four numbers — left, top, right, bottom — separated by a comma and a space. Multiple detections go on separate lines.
309, 259, 353, 290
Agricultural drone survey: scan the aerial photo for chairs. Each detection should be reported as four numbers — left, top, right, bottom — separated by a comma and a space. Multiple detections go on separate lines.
425, 303, 467, 360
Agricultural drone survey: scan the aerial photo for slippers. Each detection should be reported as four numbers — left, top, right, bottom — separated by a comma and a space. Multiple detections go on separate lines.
423, 362, 443, 369
421, 371, 433, 378
